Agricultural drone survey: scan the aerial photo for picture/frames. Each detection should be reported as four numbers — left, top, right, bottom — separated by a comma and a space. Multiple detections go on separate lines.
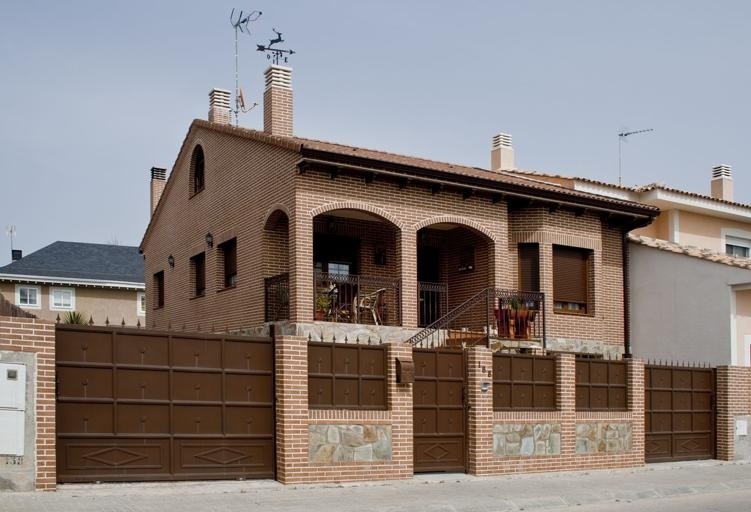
455, 246, 477, 274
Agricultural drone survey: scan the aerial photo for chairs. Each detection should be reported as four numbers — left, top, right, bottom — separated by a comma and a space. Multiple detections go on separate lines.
354, 287, 387, 324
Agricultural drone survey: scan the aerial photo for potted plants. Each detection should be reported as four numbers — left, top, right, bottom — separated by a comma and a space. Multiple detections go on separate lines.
313, 294, 331, 321
